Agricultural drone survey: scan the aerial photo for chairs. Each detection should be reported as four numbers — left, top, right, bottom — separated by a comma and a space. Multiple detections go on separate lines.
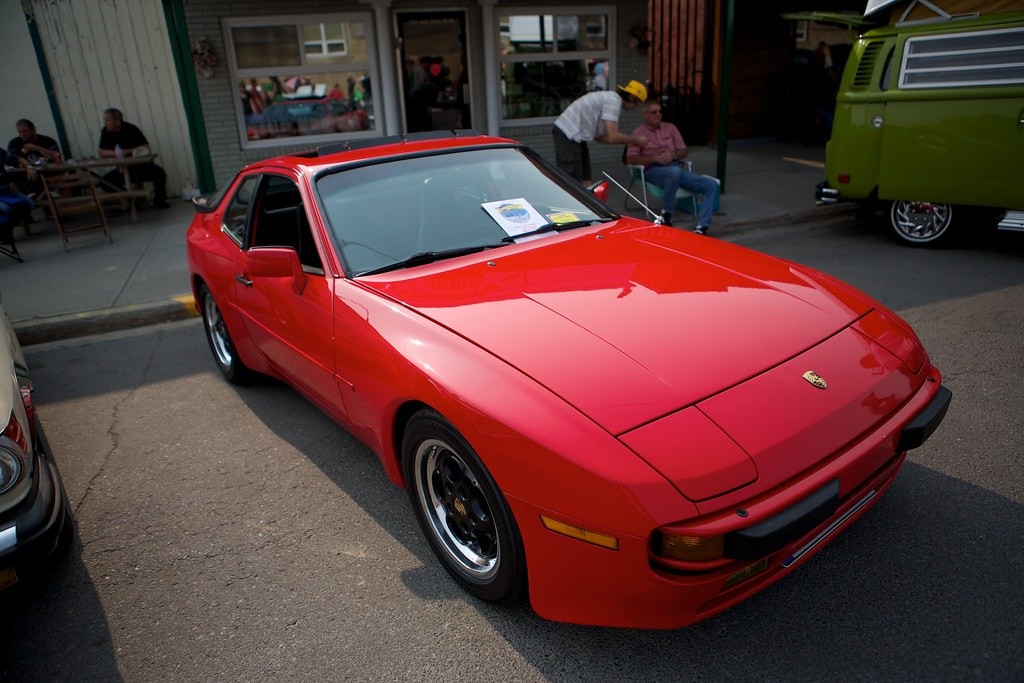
41, 172, 113, 253
0, 217, 25, 265
623, 145, 698, 222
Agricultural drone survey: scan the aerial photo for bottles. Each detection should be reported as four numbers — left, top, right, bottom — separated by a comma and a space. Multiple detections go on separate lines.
54, 151, 61, 164
115, 144, 124, 160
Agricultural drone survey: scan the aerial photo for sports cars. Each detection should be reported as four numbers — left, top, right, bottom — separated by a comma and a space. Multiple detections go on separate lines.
186, 127, 952, 628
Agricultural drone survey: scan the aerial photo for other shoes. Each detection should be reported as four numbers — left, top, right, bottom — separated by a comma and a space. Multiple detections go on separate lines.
661, 211, 674, 228
153, 200, 169, 208
692, 225, 708, 236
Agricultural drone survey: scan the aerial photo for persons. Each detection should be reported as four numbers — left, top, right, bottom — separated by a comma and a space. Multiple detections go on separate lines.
6, 118, 63, 205
0, 146, 37, 246
404, 49, 463, 132
97, 107, 172, 210
239, 68, 374, 124
551, 78, 651, 191
595, 59, 609, 91
627, 100, 721, 236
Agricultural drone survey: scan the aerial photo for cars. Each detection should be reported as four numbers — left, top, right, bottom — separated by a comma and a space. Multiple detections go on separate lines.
0, 297, 71, 591
248, 96, 369, 141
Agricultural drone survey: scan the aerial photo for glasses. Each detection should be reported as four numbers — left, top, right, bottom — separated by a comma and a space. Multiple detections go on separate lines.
648, 109, 662, 115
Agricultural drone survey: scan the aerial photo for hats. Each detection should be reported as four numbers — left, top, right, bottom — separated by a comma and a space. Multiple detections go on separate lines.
618, 80, 647, 103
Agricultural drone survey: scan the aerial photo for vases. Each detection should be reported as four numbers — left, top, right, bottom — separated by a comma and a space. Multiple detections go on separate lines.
201, 66, 214, 79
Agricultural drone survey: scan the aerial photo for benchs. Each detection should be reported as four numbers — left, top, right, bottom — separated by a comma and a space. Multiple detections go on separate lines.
253, 185, 420, 267
39, 190, 150, 209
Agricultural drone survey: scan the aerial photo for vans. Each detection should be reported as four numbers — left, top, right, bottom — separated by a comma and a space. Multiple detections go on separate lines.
784, 0, 1024, 250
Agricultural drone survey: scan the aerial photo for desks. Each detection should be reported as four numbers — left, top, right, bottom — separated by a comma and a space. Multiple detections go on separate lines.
9, 152, 157, 224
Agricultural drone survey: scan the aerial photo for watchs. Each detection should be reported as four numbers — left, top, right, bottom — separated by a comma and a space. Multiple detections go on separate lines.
26, 165, 34, 170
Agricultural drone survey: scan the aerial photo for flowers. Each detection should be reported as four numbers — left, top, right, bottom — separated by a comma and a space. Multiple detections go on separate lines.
189, 40, 216, 67
